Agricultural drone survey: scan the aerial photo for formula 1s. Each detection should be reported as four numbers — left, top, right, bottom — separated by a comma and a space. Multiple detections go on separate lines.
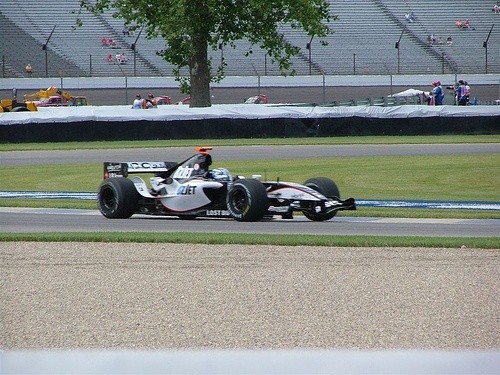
96, 145, 358, 222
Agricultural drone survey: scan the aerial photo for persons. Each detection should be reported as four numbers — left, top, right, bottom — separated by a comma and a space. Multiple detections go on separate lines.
430, 82, 441, 105
107, 54, 114, 62
456, 18, 463, 28
108, 37, 115, 45
101, 36, 108, 46
447, 35, 453, 47
187, 154, 214, 179
430, 34, 436, 44
112, 41, 118, 49
493, 4, 500, 14
116, 52, 128, 62
463, 80, 470, 105
26, 64, 32, 74
452, 79, 467, 106
426, 35, 433, 47
463, 18, 471, 29
134, 93, 158, 109
439, 37, 444, 45
436, 80, 444, 105
404, 10, 417, 23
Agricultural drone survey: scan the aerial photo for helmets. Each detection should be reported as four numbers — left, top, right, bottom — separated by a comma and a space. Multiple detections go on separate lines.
437, 80, 441, 85
432, 81, 439, 86
